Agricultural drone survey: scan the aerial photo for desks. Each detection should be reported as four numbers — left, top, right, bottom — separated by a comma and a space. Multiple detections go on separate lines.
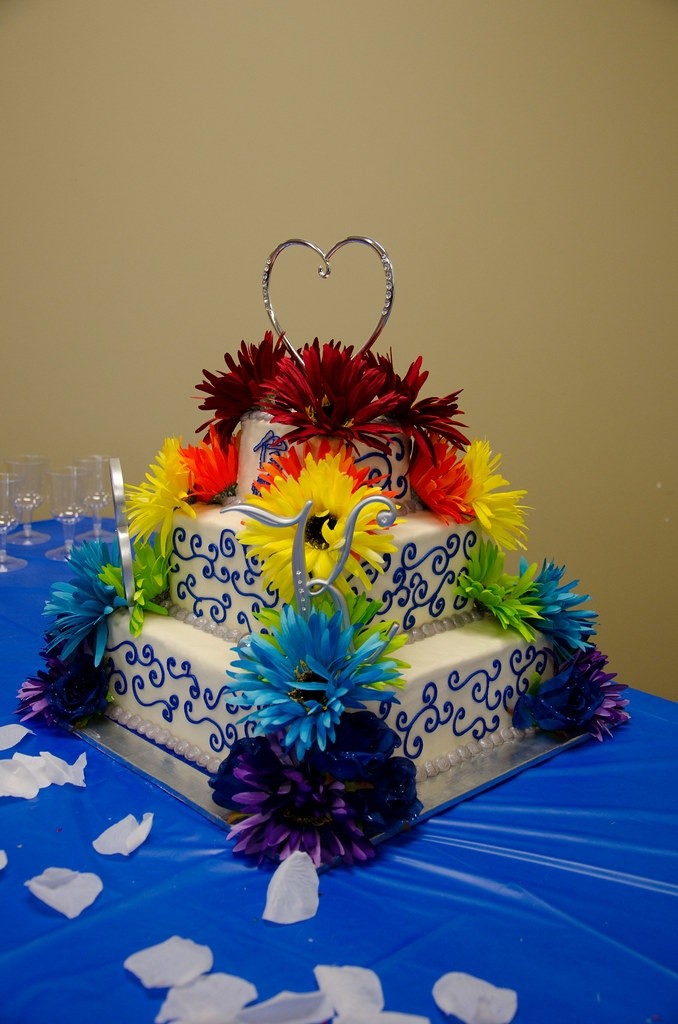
1, 508, 678, 1024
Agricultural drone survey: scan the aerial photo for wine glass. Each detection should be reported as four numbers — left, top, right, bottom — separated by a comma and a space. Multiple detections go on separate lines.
43, 464, 88, 560
0, 471, 28, 573
4, 454, 52, 545
75, 454, 115, 544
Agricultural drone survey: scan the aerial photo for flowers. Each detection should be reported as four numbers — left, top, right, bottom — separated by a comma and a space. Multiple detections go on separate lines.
12, 333, 643, 879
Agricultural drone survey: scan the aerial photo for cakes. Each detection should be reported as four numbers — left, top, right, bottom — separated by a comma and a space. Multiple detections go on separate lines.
10, 237, 633, 871
